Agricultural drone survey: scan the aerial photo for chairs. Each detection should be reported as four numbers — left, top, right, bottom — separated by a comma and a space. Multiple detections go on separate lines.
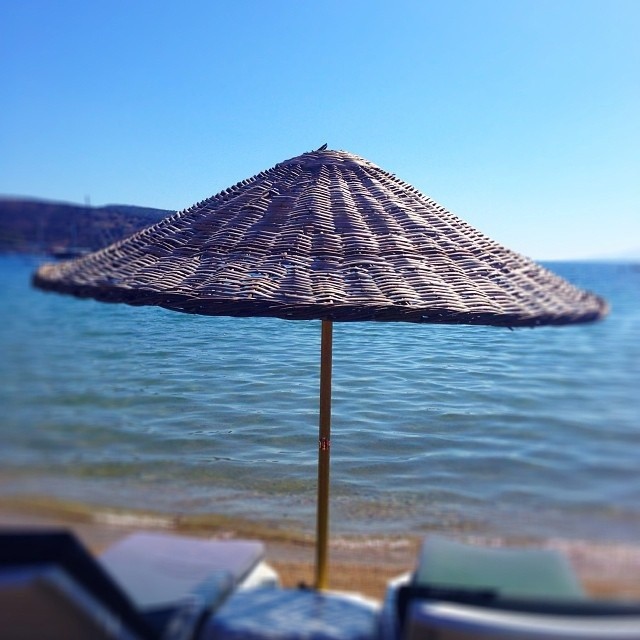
0, 524, 280, 640
385, 536, 640, 639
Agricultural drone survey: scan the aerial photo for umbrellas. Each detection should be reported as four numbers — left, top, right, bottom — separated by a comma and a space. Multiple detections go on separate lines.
31, 142, 612, 587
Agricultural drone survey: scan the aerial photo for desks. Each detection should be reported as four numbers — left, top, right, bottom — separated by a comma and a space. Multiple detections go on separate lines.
204, 589, 382, 639
